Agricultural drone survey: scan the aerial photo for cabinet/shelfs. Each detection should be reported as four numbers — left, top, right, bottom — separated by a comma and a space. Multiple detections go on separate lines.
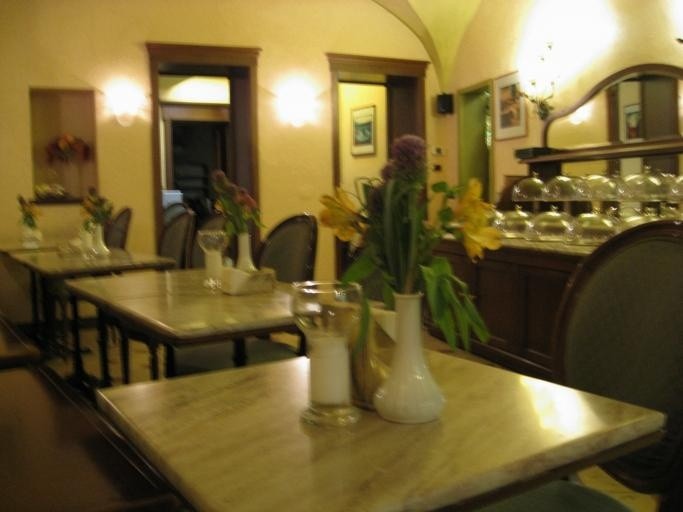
422, 231, 591, 379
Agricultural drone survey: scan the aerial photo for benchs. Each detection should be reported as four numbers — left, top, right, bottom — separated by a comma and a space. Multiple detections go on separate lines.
1, 317, 190, 512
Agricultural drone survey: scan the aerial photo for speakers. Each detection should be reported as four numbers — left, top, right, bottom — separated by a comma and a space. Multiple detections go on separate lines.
437, 95, 452, 114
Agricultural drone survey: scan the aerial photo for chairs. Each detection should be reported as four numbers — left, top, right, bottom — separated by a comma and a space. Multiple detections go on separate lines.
62, 202, 318, 376
475, 221, 683, 510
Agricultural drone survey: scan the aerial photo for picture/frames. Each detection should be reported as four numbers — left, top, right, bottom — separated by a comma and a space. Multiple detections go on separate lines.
493, 71, 528, 142
350, 104, 377, 157
622, 103, 643, 143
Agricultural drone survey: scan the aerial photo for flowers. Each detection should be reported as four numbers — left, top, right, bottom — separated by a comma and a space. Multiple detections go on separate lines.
45, 133, 92, 162
320, 137, 500, 347
34, 182, 69, 199
16, 194, 40, 226
82, 187, 115, 225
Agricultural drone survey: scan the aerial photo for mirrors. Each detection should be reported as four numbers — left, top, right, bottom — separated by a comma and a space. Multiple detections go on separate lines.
541, 62, 683, 217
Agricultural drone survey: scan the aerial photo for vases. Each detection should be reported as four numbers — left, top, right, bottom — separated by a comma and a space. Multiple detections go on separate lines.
21, 226, 42, 248
370, 293, 447, 425
62, 161, 80, 199
